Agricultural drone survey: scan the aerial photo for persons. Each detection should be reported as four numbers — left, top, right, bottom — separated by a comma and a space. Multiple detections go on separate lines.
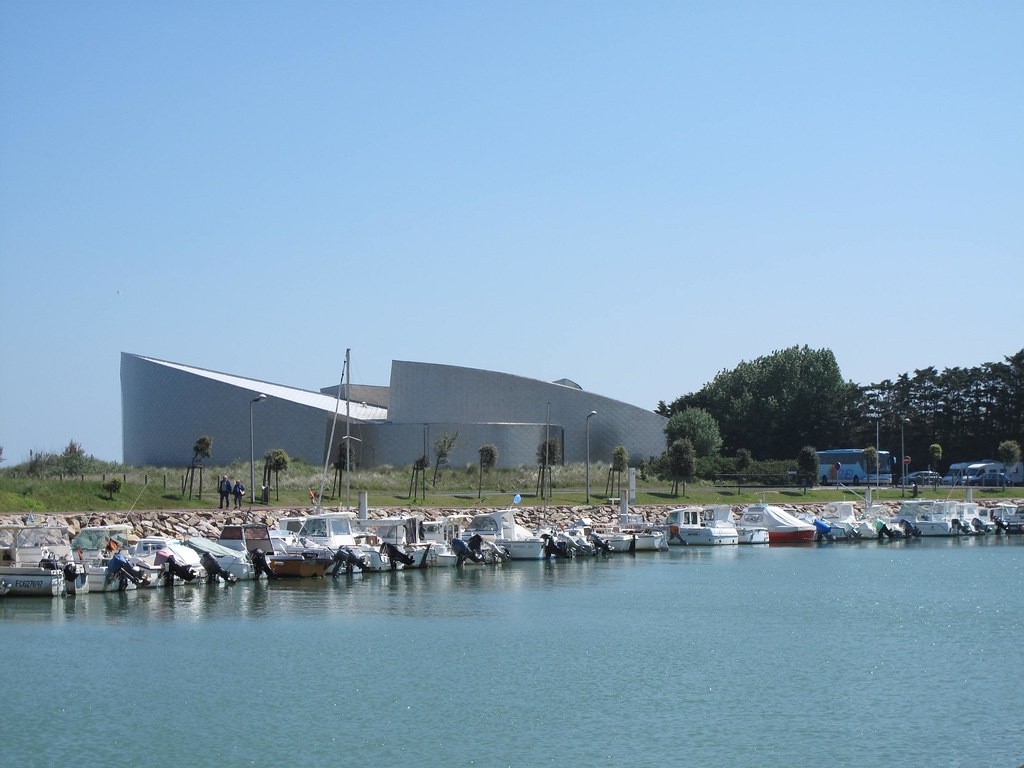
217, 475, 233, 509
232, 479, 245, 510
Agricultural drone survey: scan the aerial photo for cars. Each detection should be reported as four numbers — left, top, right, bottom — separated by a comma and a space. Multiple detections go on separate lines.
899, 471, 942, 485
967, 472, 1012, 486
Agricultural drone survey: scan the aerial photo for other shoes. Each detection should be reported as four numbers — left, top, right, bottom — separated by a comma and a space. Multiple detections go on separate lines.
233, 506, 238, 509
218, 507, 223, 509
238, 507, 242, 510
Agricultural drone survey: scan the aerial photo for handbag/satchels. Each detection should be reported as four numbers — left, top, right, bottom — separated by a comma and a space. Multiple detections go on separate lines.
240, 489, 246, 496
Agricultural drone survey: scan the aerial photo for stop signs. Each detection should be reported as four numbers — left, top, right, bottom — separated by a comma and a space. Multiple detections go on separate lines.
904, 456, 910, 464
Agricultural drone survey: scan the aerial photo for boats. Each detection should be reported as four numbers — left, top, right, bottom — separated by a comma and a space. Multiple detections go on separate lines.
0, 499, 1023, 598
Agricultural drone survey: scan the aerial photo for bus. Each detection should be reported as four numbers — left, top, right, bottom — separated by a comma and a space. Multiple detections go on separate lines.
812, 449, 897, 484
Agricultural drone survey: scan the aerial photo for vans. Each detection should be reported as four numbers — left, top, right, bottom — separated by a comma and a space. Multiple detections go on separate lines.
939, 463, 981, 485
961, 462, 1024, 485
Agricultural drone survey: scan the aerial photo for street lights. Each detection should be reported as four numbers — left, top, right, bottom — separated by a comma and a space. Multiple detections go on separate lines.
901, 418, 911, 498
586, 410, 598, 505
248, 394, 266, 503
